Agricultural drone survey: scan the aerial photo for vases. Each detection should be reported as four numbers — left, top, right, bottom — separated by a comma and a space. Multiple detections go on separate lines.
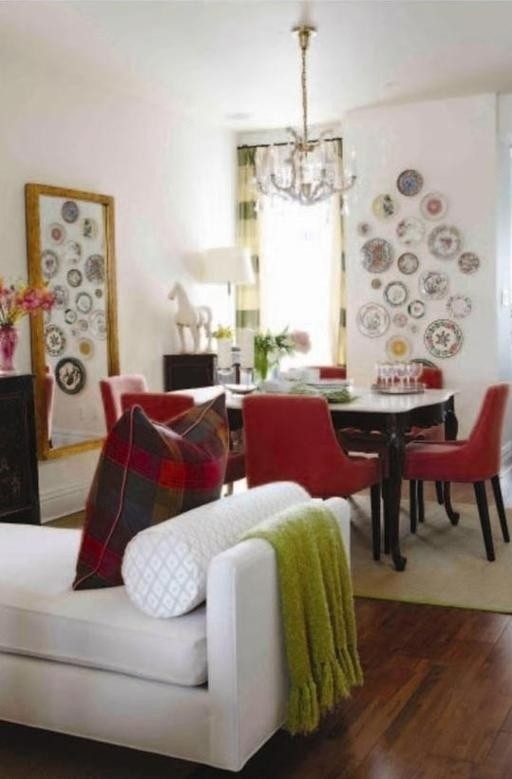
216, 337, 233, 369
0, 323, 20, 374
252, 352, 280, 386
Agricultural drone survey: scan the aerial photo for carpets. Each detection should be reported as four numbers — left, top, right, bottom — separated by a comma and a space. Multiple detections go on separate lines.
218, 479, 511, 617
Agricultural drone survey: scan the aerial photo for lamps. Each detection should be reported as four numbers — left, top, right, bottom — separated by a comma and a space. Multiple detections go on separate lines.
203, 245, 256, 328
246, 26, 365, 217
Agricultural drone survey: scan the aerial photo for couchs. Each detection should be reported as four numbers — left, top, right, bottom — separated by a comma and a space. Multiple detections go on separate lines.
2, 481, 354, 778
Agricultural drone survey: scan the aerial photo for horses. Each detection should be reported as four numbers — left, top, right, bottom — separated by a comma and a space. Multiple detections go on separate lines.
167, 281, 214, 353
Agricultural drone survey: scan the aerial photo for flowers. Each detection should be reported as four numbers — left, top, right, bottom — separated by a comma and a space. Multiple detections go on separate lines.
212, 324, 236, 340
248, 326, 316, 379
0, 275, 56, 324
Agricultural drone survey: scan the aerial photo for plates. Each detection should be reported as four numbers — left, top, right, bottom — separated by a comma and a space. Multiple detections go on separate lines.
354, 164, 479, 362
40, 201, 111, 394
222, 378, 361, 396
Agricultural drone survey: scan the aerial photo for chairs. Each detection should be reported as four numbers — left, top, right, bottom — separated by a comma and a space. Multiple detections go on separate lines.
386, 381, 510, 560
368, 364, 444, 522
242, 392, 385, 563
122, 389, 242, 500
303, 366, 348, 453
99, 372, 147, 437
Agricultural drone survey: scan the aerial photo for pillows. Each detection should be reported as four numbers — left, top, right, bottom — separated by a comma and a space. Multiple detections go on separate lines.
121, 480, 314, 617
70, 389, 233, 599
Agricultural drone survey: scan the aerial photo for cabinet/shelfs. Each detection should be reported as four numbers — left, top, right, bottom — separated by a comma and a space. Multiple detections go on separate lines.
1, 374, 42, 524
165, 353, 217, 393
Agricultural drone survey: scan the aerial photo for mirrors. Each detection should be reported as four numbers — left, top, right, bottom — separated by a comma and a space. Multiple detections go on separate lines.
24, 183, 119, 462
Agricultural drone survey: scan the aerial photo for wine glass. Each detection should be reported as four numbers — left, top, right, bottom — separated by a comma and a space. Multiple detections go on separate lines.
371, 359, 425, 395
